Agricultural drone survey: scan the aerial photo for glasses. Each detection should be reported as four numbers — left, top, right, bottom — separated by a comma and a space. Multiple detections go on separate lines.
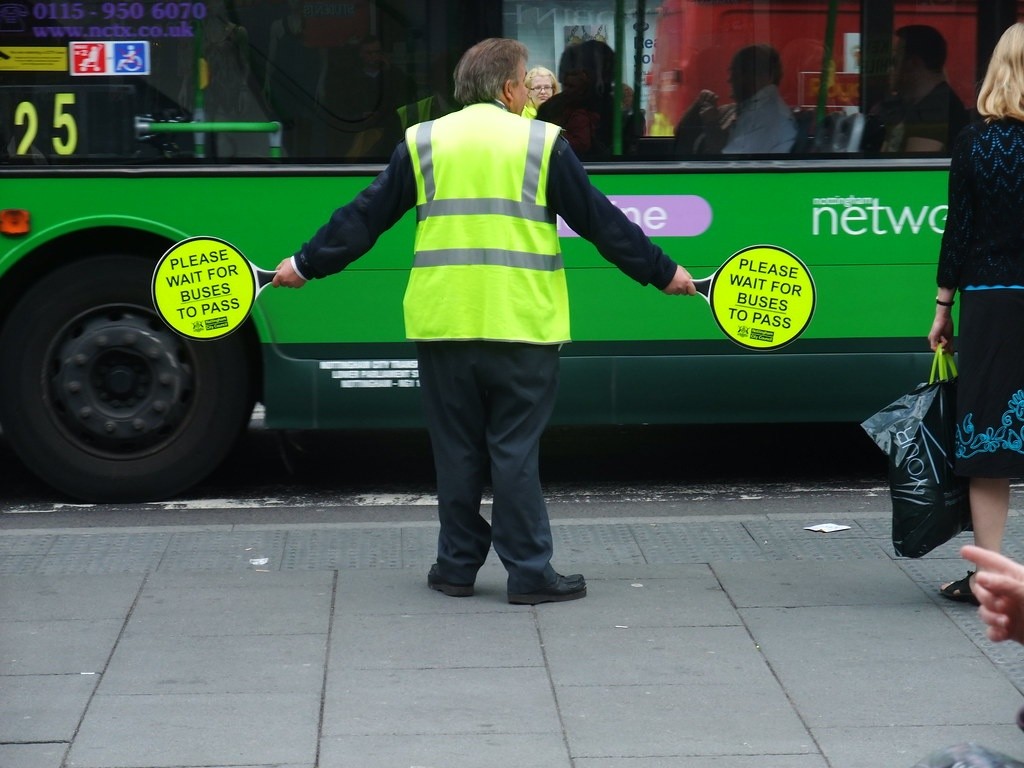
529, 87, 553, 92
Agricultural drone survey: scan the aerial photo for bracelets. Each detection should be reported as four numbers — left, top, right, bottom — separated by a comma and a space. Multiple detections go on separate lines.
936, 296, 955, 306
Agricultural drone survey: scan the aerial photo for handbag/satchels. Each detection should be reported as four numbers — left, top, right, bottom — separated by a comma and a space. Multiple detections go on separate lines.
860, 344, 974, 558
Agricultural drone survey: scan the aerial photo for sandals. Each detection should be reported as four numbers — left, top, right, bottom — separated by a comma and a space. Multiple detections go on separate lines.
939, 570, 983, 606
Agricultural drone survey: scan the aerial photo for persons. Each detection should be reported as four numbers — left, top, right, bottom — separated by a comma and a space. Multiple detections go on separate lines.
674, 43, 797, 153
1, 0, 465, 164
520, 67, 559, 121
273, 38, 696, 605
927, 21, 1024, 606
534, 40, 646, 162
843, 23, 967, 152
915, 545, 1024, 768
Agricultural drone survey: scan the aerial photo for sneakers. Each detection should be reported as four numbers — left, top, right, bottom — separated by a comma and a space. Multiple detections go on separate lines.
507, 574, 588, 604
428, 563, 473, 596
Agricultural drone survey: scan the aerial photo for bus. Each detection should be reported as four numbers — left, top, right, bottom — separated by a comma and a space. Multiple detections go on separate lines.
1, 0, 1024, 504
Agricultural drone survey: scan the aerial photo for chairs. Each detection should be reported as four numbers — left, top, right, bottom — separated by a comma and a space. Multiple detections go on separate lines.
591, 93, 982, 159
